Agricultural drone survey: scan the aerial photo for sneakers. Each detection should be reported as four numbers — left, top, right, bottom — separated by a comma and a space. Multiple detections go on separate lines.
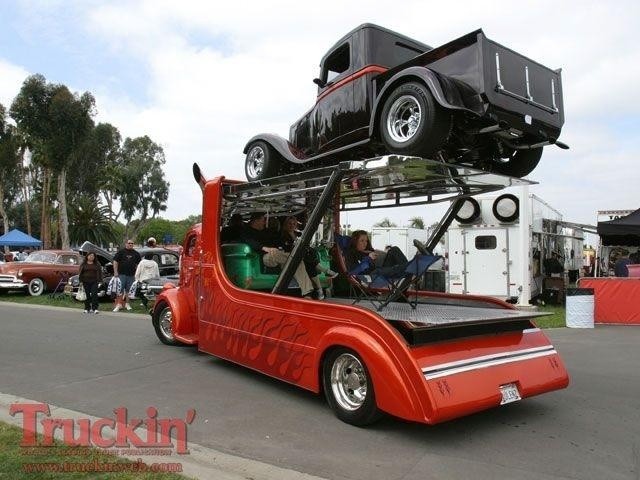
413, 239, 429, 256
113, 304, 122, 311
317, 288, 325, 300
325, 269, 339, 278
84, 309, 87, 313
125, 303, 132, 310
95, 310, 99, 314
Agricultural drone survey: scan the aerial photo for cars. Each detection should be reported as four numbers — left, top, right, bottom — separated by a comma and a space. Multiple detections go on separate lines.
1, 250, 84, 298
66, 241, 180, 302
139, 275, 180, 306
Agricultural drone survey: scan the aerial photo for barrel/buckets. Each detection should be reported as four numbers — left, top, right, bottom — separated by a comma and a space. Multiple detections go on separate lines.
565, 288, 595, 328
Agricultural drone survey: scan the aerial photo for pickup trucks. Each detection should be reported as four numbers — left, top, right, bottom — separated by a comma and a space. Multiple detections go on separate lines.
242, 20, 571, 181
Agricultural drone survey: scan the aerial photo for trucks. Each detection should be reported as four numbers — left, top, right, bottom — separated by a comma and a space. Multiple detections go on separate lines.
149, 155, 573, 426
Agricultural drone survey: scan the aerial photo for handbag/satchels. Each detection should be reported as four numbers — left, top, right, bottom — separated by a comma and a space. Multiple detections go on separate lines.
384, 247, 408, 266
106, 277, 121, 296
128, 281, 139, 299
76, 290, 87, 301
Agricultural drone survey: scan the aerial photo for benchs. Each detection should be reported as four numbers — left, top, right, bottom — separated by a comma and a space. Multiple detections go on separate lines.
222, 242, 335, 297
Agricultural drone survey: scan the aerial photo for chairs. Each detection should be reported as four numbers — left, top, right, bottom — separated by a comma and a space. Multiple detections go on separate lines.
335, 232, 446, 311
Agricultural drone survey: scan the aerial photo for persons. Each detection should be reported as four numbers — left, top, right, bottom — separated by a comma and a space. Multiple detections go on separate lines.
0, 246, 38, 263
221, 214, 246, 244
79, 253, 102, 314
276, 216, 340, 302
238, 212, 315, 301
342, 228, 432, 280
134, 250, 162, 314
615, 249, 634, 278
112, 240, 141, 313
